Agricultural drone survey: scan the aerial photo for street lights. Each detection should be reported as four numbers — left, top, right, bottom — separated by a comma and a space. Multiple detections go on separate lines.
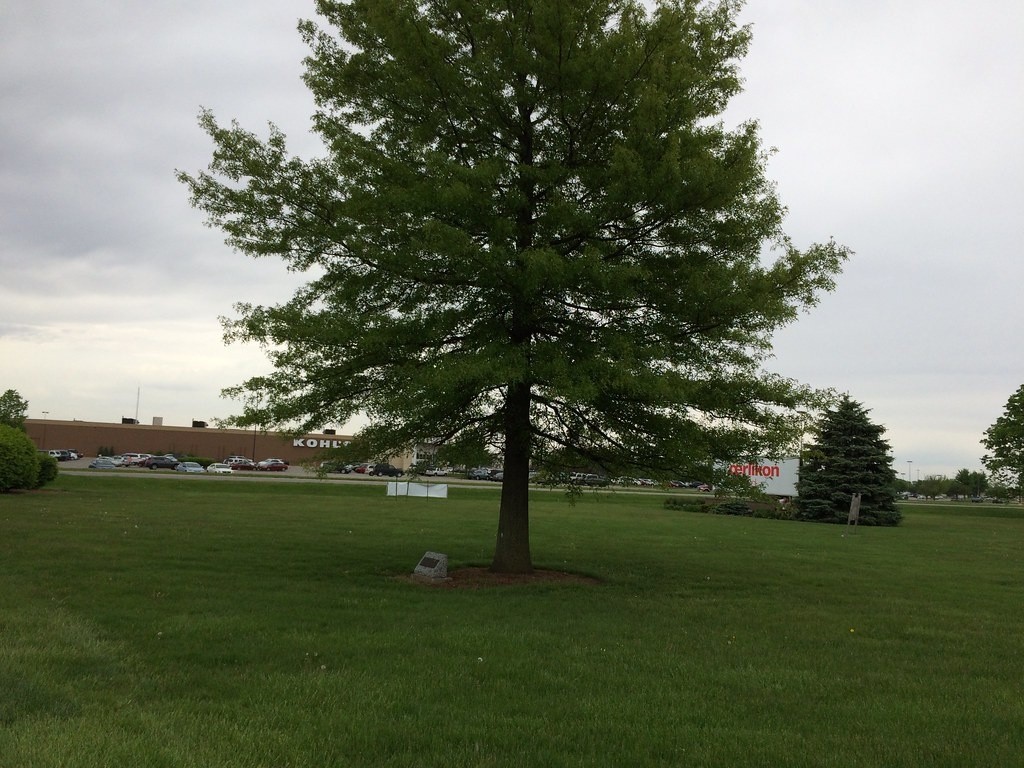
42, 411, 50, 418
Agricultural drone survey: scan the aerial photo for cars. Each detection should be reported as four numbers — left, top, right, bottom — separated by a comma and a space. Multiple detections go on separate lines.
319, 460, 730, 495
50, 447, 289, 475
